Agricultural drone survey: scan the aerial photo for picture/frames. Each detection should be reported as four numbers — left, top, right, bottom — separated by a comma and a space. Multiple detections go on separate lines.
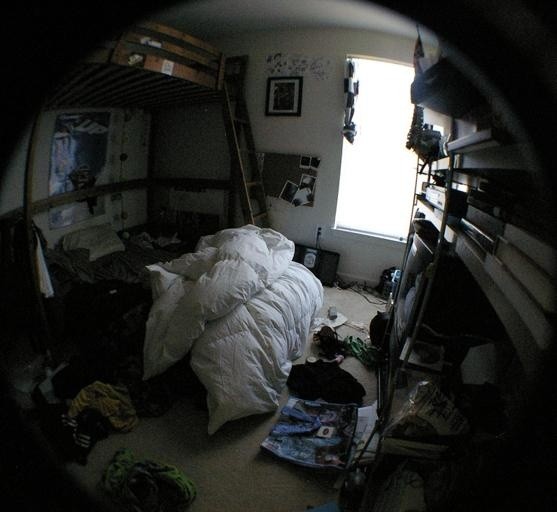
265, 76, 303, 117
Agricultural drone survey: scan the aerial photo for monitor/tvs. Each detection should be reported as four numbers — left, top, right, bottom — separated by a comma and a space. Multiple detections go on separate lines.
390, 229, 435, 350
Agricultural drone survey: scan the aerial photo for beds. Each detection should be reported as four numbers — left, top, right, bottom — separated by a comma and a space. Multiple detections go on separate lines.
1, 18, 271, 326
338, 116, 557, 512
